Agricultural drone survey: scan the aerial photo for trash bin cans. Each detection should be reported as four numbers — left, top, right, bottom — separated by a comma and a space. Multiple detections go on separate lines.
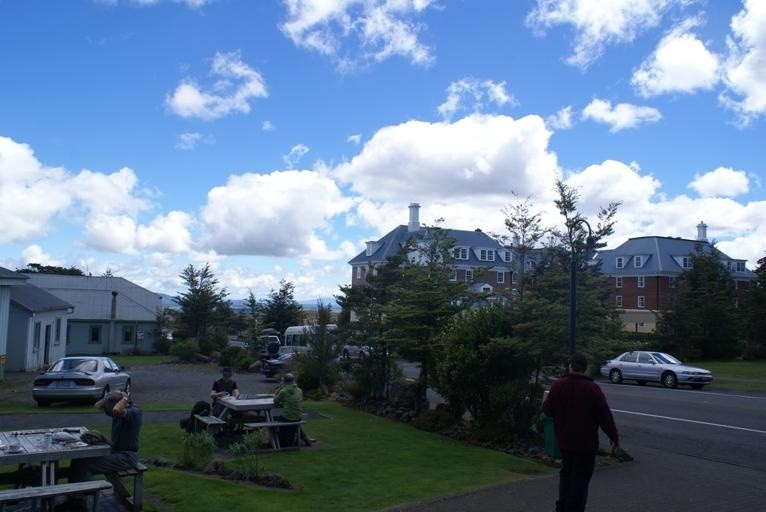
539, 412, 563, 458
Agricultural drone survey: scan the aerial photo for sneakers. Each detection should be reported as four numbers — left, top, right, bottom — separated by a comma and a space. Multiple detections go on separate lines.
54, 500, 89, 512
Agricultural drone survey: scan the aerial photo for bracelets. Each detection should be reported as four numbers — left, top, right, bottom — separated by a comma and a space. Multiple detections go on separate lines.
122, 396, 128, 401
544, 390, 550, 393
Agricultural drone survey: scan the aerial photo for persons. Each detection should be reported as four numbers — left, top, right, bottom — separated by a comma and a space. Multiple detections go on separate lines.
540, 353, 619, 512
263, 372, 317, 449
55, 389, 143, 512
206, 368, 240, 435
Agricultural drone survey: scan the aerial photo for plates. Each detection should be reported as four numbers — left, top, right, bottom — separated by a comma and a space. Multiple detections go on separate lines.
7, 447, 24, 453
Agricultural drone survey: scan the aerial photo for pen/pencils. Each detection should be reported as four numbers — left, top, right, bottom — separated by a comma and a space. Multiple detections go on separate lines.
65, 440, 80, 443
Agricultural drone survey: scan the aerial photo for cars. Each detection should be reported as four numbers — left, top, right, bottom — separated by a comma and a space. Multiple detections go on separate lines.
29, 354, 134, 410
596, 349, 714, 393
244, 335, 282, 356
256, 352, 341, 386
332, 342, 393, 367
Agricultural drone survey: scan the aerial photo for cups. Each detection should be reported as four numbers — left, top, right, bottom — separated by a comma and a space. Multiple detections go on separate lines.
43, 432, 52, 449
9, 445, 20, 450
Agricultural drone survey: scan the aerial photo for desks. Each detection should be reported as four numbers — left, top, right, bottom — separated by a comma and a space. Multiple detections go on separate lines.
216, 392, 283, 451
0, 425, 131, 511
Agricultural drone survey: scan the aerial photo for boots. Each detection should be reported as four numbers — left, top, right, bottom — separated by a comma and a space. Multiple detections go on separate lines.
303, 435, 316, 445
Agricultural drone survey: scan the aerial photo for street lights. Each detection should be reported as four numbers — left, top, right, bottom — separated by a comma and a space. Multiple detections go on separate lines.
566, 216, 597, 377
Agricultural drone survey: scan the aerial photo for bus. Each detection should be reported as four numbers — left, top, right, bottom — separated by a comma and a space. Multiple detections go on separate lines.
275, 325, 344, 361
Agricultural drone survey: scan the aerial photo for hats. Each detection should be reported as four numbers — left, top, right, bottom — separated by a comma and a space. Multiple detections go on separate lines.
103, 391, 132, 407
568, 351, 587, 363
282, 372, 294, 385
222, 367, 233, 376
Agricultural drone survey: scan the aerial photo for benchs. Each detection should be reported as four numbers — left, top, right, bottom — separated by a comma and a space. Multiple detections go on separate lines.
115, 460, 148, 511
0, 478, 112, 511
192, 412, 307, 454
